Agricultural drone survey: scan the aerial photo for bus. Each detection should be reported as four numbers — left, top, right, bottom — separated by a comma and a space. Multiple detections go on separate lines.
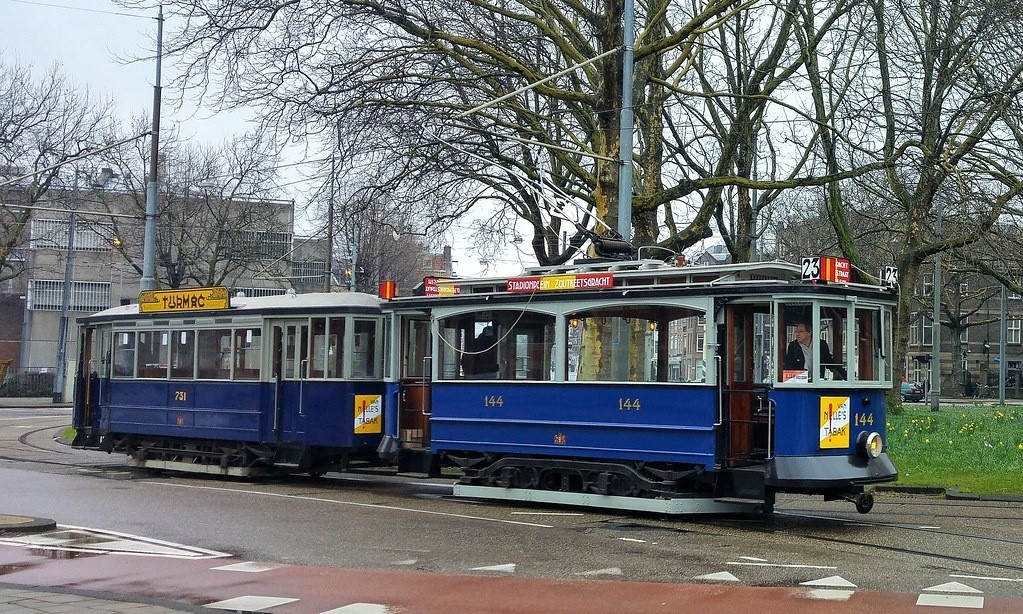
70, 121, 906, 516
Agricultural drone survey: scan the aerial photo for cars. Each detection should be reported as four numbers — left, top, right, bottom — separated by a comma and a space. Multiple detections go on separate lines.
899, 381, 924, 403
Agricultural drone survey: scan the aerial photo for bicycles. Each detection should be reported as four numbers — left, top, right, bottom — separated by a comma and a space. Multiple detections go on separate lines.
972, 382, 996, 399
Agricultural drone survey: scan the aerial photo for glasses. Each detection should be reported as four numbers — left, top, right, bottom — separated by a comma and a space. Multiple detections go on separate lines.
793, 331, 807, 334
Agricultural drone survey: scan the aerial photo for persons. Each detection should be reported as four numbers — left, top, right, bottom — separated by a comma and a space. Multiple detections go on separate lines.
462, 326, 506, 379
784, 320, 846, 382
902, 375, 906, 382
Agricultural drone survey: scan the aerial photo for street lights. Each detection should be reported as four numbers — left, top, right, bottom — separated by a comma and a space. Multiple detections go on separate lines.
48, 170, 122, 402
